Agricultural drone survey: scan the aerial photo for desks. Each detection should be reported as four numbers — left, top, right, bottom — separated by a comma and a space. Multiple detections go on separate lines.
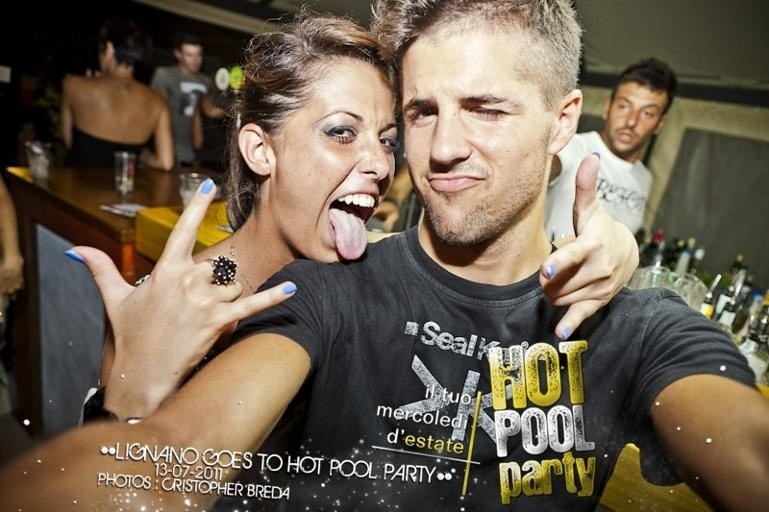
0, 153, 237, 438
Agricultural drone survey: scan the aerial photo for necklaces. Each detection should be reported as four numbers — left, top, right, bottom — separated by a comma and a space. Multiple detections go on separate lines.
229, 229, 256, 295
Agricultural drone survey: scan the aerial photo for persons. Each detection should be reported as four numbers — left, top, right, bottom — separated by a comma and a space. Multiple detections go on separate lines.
63, 13, 643, 436
52, 18, 178, 173
0, 1, 768, 510
0, 178, 28, 415
150, 34, 229, 169
546, 55, 679, 264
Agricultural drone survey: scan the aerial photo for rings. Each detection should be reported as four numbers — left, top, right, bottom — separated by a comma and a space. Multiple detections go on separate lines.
203, 251, 237, 287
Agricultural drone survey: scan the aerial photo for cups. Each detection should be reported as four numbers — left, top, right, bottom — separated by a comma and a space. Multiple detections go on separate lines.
179, 171, 208, 214
23, 142, 55, 178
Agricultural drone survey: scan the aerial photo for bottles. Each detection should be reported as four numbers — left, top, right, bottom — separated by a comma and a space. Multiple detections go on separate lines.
635, 227, 769, 394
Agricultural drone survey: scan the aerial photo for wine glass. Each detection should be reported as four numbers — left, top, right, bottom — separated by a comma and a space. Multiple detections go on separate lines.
113, 150, 138, 217
